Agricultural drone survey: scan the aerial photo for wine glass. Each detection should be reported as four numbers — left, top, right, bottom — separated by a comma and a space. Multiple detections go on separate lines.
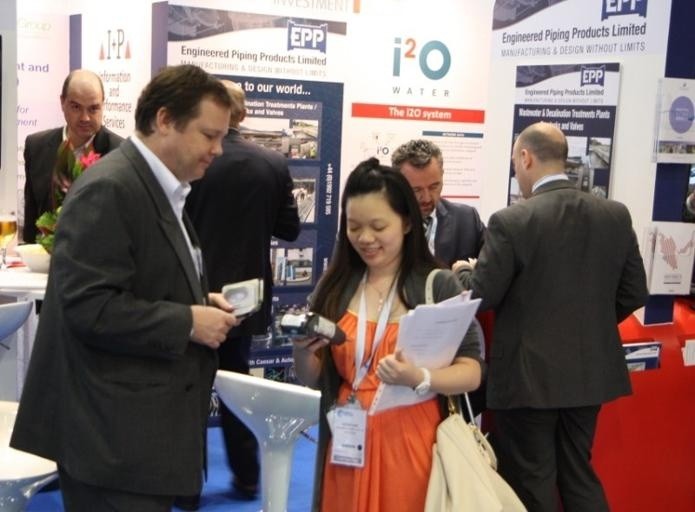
0, 210, 18, 272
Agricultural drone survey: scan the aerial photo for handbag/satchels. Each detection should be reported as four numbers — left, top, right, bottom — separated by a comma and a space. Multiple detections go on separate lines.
424, 413, 528, 512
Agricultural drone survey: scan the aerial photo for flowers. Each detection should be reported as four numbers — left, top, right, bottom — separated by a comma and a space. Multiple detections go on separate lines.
32, 136, 103, 255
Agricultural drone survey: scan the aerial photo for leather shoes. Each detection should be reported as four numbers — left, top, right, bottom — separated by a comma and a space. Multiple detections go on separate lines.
230, 479, 261, 502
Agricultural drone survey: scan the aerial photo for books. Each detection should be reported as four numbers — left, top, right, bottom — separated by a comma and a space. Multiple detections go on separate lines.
622, 340, 664, 373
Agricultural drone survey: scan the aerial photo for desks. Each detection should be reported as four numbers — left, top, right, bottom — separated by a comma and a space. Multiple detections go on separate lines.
0, 267, 49, 403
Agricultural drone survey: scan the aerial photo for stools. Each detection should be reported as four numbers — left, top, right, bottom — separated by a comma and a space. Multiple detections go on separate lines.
214, 370, 322, 511
